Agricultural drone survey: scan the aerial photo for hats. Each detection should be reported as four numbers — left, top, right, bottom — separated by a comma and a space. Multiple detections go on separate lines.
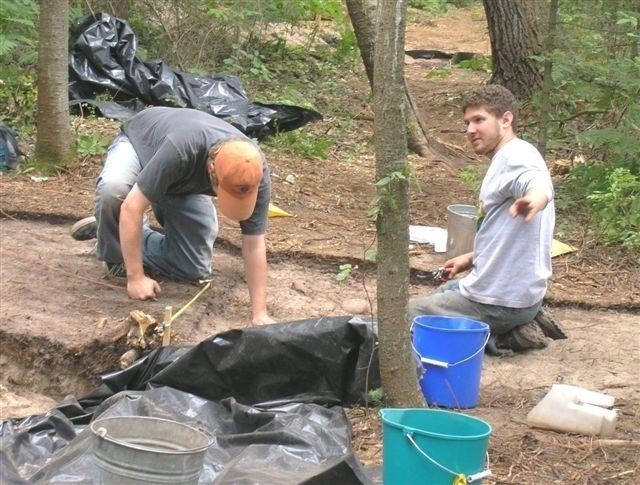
213, 140, 264, 222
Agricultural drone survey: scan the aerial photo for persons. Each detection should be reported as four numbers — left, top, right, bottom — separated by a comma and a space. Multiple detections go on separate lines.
71, 106, 278, 328
408, 83, 569, 356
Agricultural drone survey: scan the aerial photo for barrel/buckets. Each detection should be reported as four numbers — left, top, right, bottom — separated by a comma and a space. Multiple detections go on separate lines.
380, 406, 491, 485
445, 204, 478, 260
410, 314, 490, 409
89, 416, 216, 485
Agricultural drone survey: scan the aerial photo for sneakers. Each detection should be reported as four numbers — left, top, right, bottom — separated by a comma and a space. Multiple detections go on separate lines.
102, 260, 129, 283
71, 214, 98, 241
496, 306, 569, 351
